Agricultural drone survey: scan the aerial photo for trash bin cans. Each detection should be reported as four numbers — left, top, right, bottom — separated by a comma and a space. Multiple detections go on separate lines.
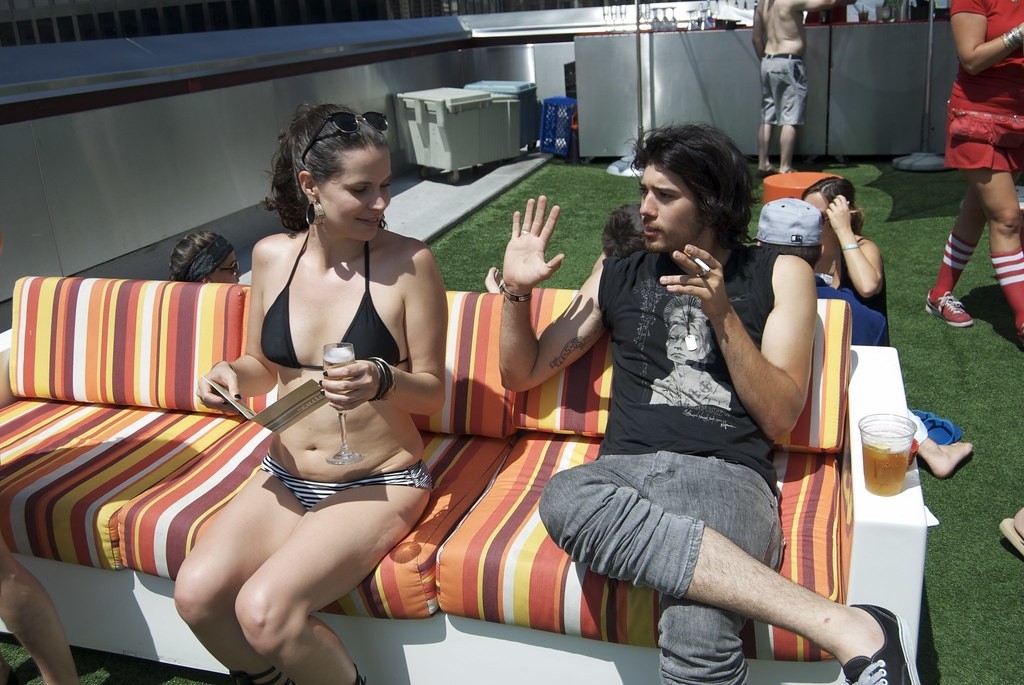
393, 88, 522, 187
464, 79, 538, 153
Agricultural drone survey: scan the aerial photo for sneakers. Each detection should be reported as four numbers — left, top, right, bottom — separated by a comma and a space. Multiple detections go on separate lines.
925, 289, 973, 327
845, 604, 921, 685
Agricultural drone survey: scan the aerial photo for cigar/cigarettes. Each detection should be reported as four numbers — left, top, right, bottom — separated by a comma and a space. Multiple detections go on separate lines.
684, 250, 711, 272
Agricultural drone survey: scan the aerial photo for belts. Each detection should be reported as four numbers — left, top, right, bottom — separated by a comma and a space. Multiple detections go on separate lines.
763, 54, 803, 59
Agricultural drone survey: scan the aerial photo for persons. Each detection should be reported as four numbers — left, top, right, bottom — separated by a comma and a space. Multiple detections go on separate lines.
174, 99, 448, 685
499, 118, 921, 685
0, 531, 82, 685
800, 175, 940, 527
999, 506, 1024, 556
752, 0, 856, 177
924, 0, 1024, 341
750, 196, 974, 479
171, 230, 242, 284
591, 204, 648, 273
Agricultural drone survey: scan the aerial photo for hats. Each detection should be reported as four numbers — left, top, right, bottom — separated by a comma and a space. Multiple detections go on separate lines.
757, 197, 823, 246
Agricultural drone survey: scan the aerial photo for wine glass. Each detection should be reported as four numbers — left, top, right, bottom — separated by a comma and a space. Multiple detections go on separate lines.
322, 343, 365, 466
600, 0, 719, 30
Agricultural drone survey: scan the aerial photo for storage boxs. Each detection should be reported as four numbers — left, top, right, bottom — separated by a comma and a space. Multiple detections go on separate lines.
537, 96, 577, 157
464, 81, 538, 151
565, 61, 577, 98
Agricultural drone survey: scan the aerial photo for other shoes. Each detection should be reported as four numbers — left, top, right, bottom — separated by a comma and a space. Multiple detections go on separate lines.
230, 667, 294, 685
778, 168, 798, 174
757, 165, 778, 178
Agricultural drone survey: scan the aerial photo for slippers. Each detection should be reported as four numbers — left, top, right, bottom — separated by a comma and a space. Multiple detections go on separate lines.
923, 417, 962, 445
910, 410, 934, 422
1000, 518, 1024, 555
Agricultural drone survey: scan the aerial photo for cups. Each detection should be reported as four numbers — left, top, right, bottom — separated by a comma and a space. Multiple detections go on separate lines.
858, 9, 869, 22
859, 413, 918, 498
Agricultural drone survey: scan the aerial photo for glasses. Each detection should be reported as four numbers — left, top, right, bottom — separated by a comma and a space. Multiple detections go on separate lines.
221, 260, 240, 273
302, 112, 388, 165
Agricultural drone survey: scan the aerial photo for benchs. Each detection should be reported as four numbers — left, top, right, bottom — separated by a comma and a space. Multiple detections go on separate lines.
0, 276, 928, 685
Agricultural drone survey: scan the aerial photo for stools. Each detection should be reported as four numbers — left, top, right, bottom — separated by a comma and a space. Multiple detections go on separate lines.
763, 172, 844, 207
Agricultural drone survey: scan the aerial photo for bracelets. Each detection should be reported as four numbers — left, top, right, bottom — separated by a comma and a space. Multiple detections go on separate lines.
841, 244, 860, 250
210, 360, 234, 371
502, 284, 532, 301
1000, 25, 1024, 53
364, 357, 397, 401
